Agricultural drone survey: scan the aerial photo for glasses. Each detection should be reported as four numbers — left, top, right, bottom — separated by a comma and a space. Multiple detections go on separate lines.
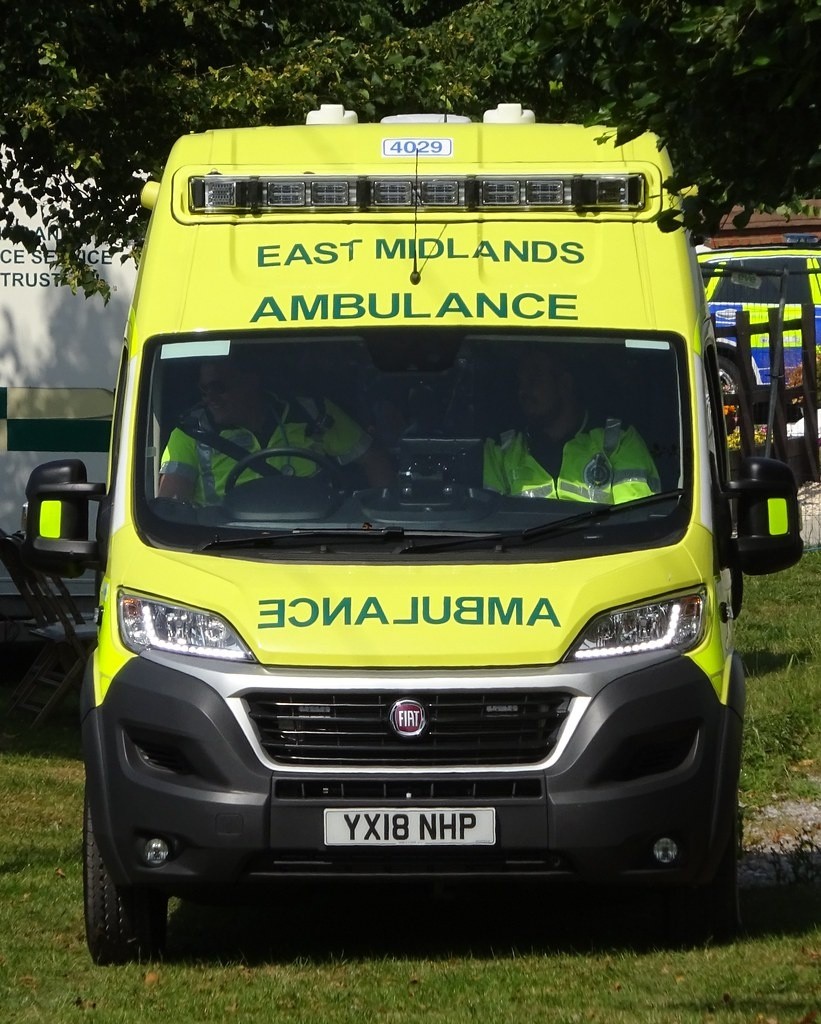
198, 380, 249, 398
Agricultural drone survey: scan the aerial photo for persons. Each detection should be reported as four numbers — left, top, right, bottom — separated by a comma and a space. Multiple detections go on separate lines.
480, 342, 661, 507
155, 348, 380, 506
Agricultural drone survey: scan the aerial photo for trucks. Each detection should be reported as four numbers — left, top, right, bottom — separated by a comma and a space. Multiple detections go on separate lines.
20, 104, 804, 966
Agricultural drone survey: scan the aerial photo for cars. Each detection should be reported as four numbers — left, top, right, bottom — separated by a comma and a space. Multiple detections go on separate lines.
696, 233, 821, 436
